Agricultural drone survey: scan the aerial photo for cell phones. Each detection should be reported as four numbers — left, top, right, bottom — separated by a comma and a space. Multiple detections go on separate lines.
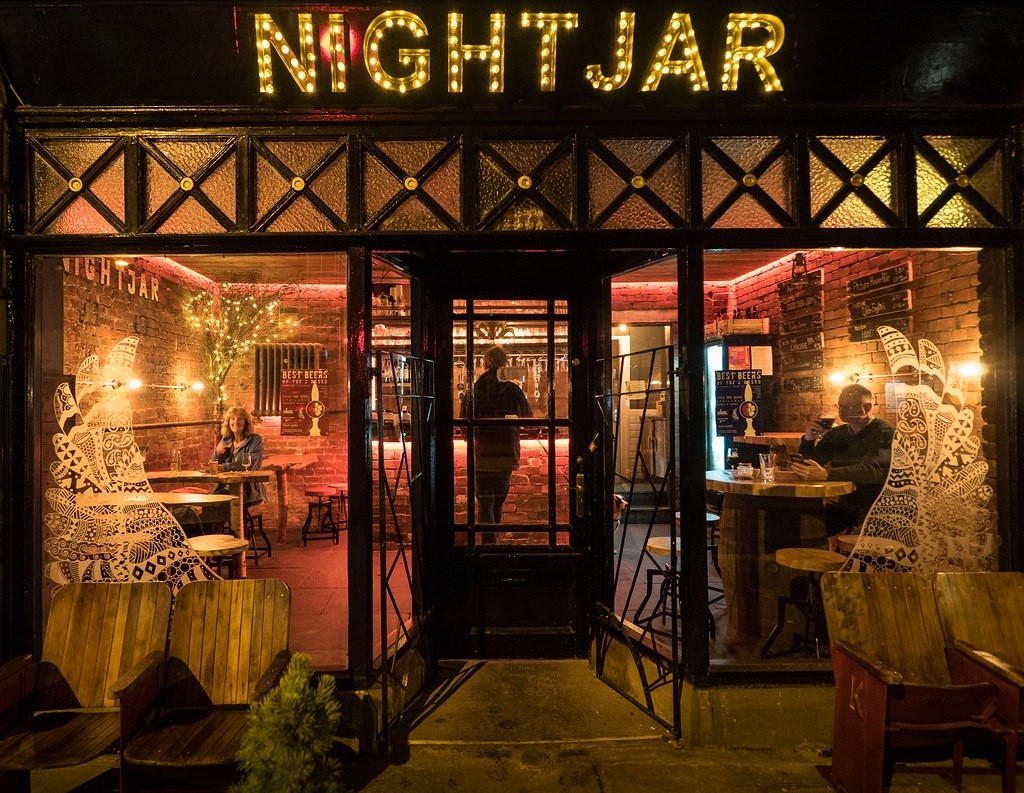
789, 453, 803, 465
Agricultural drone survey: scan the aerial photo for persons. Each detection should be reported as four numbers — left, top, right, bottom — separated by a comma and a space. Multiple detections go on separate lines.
459, 345, 534, 546
201, 406, 264, 562
791, 384, 896, 535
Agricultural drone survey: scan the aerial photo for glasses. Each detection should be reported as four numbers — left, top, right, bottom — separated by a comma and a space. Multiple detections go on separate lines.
834, 403, 864, 413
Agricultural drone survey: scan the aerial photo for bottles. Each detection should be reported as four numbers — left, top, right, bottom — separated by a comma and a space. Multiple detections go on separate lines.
171, 450, 179, 477
733, 307, 742, 324
737, 463, 753, 479
746, 307, 758, 319
717, 308, 729, 321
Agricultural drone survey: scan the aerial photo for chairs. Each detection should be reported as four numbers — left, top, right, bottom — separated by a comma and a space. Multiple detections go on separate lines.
0, 578, 292, 793
821, 570, 1024, 793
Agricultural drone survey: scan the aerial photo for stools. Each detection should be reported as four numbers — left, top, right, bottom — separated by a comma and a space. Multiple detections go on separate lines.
180, 534, 249, 581
240, 501, 272, 567
302, 483, 348, 546
632, 510, 906, 658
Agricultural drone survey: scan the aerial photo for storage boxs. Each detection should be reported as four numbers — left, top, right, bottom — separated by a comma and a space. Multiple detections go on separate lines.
704, 316, 770, 340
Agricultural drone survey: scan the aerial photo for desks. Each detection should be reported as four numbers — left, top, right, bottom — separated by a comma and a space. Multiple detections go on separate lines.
706, 467, 857, 653
68, 467, 273, 580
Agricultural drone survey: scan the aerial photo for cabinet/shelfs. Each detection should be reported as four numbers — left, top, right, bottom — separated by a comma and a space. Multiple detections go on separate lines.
636, 415, 669, 481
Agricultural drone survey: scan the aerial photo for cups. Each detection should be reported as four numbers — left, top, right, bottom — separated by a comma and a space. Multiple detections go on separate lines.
759, 453, 777, 482
753, 469, 760, 481
818, 418, 836, 429
209, 459, 218, 475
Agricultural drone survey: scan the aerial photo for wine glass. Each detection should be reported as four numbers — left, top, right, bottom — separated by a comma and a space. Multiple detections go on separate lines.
454, 349, 569, 373
241, 454, 252, 475
